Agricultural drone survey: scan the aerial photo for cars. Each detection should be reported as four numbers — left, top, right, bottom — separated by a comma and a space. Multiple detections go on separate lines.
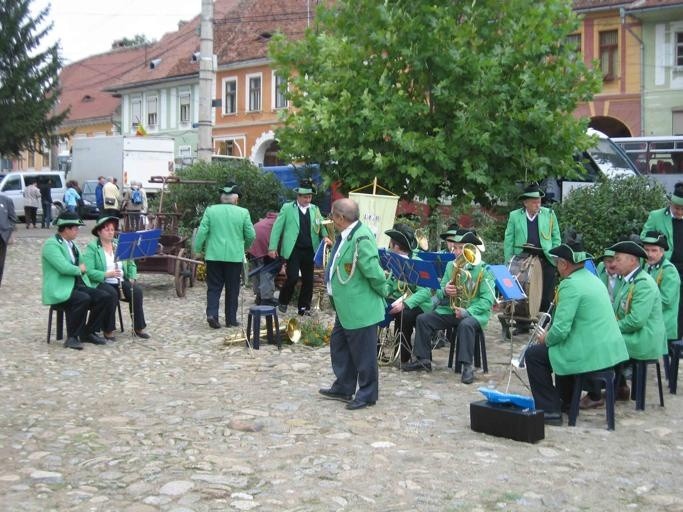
79, 180, 102, 218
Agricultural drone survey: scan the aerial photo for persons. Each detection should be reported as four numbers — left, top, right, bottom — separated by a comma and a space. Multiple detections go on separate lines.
0, 193, 17, 288
194, 183, 256, 329
21, 175, 148, 233
318, 197, 390, 410
637, 181, 683, 358
81, 215, 151, 341
267, 182, 333, 316
384, 230, 681, 427
502, 183, 561, 336
40, 211, 113, 349
246, 211, 283, 306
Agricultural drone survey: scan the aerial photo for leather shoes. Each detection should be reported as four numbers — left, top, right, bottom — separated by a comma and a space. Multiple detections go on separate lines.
462, 368, 474, 384
400, 359, 432, 372
63, 329, 151, 350
575, 394, 606, 411
206, 315, 221, 329
612, 386, 632, 402
533, 409, 566, 427
344, 397, 377, 409
317, 387, 354, 402
253, 296, 311, 317
225, 322, 239, 328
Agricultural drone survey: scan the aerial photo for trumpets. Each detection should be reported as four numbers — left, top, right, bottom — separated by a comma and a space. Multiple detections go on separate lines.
222, 318, 300, 344
320, 220, 334, 271
510, 303, 555, 370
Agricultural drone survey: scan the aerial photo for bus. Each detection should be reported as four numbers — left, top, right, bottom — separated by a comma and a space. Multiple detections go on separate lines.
611, 134, 683, 201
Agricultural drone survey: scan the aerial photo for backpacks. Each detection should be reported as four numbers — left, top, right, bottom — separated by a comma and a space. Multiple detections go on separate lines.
130, 187, 142, 204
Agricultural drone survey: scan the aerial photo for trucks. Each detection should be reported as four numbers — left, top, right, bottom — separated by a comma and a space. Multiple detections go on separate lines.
58, 135, 175, 202
412, 127, 653, 207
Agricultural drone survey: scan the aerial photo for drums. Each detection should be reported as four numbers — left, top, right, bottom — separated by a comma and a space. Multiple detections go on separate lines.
507, 254, 542, 318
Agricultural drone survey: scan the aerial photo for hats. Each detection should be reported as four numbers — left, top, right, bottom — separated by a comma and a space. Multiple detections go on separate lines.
293, 178, 318, 196
385, 223, 419, 253
92, 214, 120, 236
438, 223, 460, 239
665, 182, 683, 207
518, 185, 545, 200
218, 182, 242, 199
52, 212, 87, 227
450, 230, 483, 246
549, 232, 669, 265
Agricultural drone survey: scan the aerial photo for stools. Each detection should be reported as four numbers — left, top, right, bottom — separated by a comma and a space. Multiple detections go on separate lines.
665, 339, 682, 395
448, 324, 488, 373
614, 357, 664, 411
106, 287, 132, 333
247, 304, 282, 350
46, 305, 70, 346
569, 367, 618, 430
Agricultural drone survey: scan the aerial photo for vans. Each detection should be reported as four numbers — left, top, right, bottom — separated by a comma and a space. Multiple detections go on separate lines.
0, 169, 68, 221
57, 149, 69, 170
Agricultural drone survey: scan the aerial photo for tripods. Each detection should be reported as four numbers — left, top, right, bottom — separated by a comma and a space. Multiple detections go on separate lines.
376, 307, 432, 376
433, 329, 452, 350
224, 283, 255, 359
123, 286, 156, 351
493, 317, 533, 398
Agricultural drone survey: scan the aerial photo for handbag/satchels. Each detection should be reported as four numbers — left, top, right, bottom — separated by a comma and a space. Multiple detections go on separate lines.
104, 197, 116, 205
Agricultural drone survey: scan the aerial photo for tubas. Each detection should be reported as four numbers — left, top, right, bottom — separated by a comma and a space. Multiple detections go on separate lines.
448, 243, 483, 313
